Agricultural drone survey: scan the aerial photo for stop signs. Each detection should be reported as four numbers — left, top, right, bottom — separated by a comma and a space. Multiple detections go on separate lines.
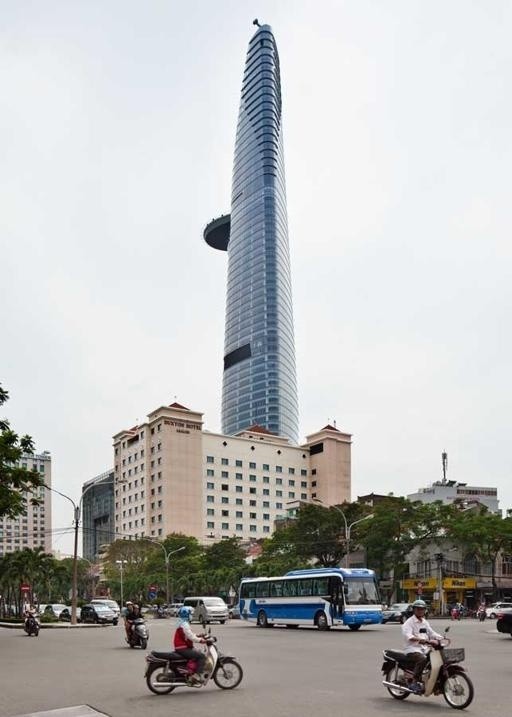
149, 583, 157, 591
21, 583, 30, 593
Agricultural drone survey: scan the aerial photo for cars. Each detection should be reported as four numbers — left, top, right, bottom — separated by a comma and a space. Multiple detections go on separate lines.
496, 609, 511, 635
3, 597, 121, 625
228, 601, 241, 619
380, 601, 429, 624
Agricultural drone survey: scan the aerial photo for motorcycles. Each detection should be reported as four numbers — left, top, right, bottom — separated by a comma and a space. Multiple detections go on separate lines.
23, 609, 42, 635
380, 624, 474, 709
142, 625, 243, 695
124, 616, 150, 649
450, 607, 461, 620
478, 607, 487, 621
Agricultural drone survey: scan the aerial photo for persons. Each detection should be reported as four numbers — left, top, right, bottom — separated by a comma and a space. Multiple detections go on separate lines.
173, 603, 215, 681
401, 599, 447, 692
454, 603, 465, 617
477, 603, 486, 617
125, 604, 148, 639
123, 600, 134, 633
24, 605, 40, 628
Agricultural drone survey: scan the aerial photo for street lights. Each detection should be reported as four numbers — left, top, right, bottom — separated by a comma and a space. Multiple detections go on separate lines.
115, 559, 128, 607
41, 477, 129, 625
311, 496, 375, 568
146, 536, 186, 602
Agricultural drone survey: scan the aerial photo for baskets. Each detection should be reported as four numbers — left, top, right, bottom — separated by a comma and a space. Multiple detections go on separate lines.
443, 647, 465, 662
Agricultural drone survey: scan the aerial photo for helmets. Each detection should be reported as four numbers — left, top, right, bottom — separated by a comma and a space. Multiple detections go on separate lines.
126, 601, 139, 609
178, 606, 194, 618
412, 599, 427, 609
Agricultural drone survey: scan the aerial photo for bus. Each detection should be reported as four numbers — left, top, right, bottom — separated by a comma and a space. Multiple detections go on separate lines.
238, 565, 384, 631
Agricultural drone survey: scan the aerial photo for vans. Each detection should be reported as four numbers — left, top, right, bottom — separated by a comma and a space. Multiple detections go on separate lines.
476, 601, 512, 618
141, 602, 186, 618
183, 596, 230, 624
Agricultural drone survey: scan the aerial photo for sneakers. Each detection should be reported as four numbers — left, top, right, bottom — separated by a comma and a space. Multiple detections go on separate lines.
192, 673, 206, 682
409, 683, 421, 690
